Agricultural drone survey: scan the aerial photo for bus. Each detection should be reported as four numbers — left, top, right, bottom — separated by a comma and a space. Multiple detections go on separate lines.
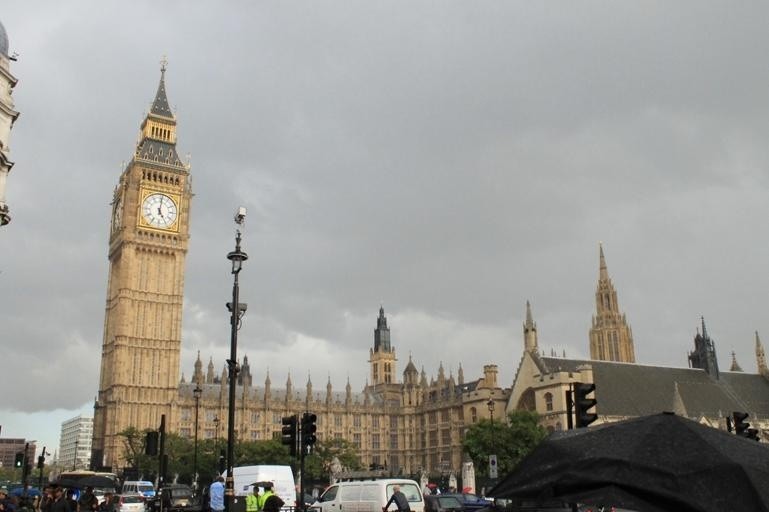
59, 469, 119, 505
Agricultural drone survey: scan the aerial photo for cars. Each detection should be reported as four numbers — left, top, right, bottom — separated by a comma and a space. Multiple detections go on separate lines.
6, 488, 41, 512
423, 493, 466, 512
295, 491, 316, 509
156, 488, 162, 499
99, 493, 149, 512
448, 490, 494, 511
161, 482, 195, 512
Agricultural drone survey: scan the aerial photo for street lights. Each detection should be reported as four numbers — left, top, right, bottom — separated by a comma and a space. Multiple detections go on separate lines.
302, 411, 318, 454
279, 414, 297, 459
213, 413, 221, 469
191, 380, 203, 485
223, 202, 248, 509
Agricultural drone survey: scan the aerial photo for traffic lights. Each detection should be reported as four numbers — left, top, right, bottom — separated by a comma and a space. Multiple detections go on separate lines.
13, 451, 24, 467
734, 411, 748, 435
748, 427, 760, 442
486, 396, 497, 493
72, 440, 79, 470
37, 455, 46, 468
571, 378, 599, 429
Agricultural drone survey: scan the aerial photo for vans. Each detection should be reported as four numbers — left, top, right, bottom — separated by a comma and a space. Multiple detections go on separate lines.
221, 464, 297, 512
121, 480, 155, 501
307, 478, 426, 511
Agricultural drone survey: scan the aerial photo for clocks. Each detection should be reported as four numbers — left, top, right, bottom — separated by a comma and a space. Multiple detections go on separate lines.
141, 192, 177, 229
114, 199, 121, 228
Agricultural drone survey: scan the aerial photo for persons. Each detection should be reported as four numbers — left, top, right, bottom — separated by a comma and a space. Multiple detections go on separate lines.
246, 487, 262, 512
423, 483, 456, 497
258, 485, 285, 512
207, 477, 226, 512
384, 485, 410, 512
0, 483, 122, 512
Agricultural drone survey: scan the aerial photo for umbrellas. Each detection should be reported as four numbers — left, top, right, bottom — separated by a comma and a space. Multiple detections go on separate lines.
249, 482, 273, 488
463, 487, 472, 494
